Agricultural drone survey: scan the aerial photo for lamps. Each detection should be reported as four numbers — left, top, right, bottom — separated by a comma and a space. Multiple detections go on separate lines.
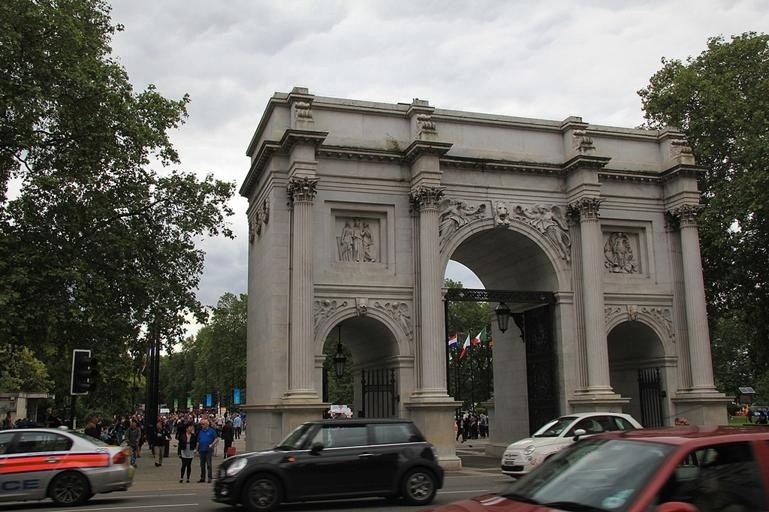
494, 302, 524, 343
333, 324, 346, 379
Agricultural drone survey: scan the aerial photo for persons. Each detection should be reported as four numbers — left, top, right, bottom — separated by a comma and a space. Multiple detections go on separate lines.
85, 410, 245, 467
674, 416, 699, 467
516, 205, 571, 263
340, 220, 377, 263
613, 232, 629, 270
177, 423, 197, 483
454, 412, 489, 443
194, 420, 219, 483
3, 411, 15, 430
44, 408, 61, 428
439, 200, 486, 254
744, 408, 769, 424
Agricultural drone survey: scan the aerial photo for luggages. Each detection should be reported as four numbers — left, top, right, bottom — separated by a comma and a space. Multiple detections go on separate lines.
226, 442, 236, 458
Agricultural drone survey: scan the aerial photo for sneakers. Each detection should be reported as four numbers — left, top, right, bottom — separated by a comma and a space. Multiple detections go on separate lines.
130, 461, 213, 484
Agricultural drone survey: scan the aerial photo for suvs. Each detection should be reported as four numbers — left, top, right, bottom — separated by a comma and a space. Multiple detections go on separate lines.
211, 417, 446, 512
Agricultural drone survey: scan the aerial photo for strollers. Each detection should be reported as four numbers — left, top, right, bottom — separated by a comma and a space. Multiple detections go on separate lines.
120, 439, 137, 468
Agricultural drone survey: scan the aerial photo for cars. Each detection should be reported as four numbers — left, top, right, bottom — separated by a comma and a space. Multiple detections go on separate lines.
500, 412, 648, 478
753, 406, 769, 416
422, 424, 769, 511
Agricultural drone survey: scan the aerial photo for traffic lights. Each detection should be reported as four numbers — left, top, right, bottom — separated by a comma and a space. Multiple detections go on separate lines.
71, 349, 96, 396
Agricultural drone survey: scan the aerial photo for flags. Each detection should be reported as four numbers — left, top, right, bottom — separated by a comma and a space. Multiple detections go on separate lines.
449, 334, 458, 348
459, 332, 471, 360
471, 326, 487, 346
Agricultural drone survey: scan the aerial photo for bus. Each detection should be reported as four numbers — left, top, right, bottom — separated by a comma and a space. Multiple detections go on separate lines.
0, 427, 133, 507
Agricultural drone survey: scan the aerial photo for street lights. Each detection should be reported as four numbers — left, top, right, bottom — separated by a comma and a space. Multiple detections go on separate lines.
197, 402, 204, 422
190, 402, 194, 421
